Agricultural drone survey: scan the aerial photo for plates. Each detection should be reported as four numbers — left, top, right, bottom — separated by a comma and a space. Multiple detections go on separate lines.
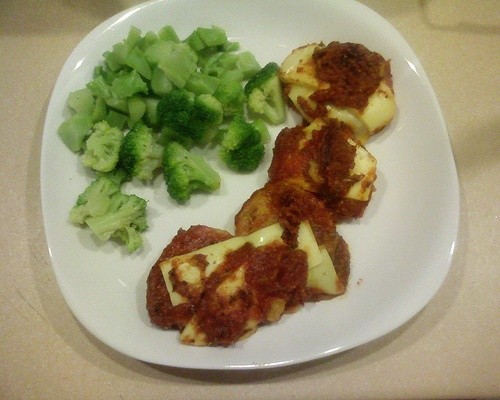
40, 1, 461, 372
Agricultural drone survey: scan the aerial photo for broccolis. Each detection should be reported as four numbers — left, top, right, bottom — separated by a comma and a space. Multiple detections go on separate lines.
57, 22, 283, 254
